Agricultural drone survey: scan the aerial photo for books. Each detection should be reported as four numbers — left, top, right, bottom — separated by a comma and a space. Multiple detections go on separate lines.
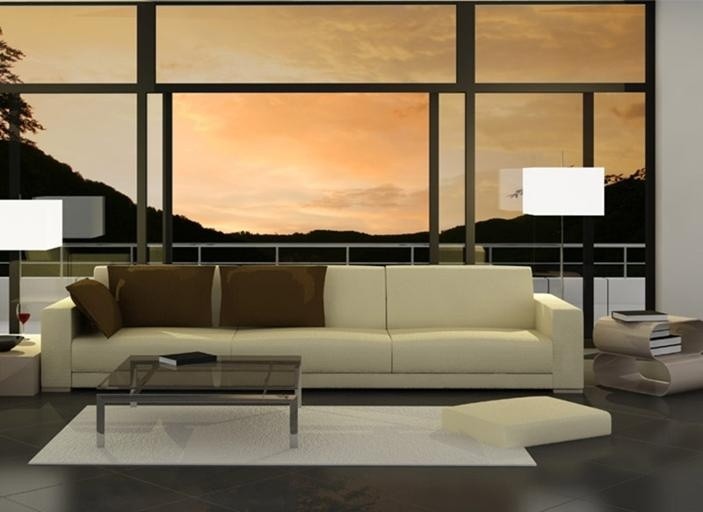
159, 352, 217, 366
611, 310, 682, 356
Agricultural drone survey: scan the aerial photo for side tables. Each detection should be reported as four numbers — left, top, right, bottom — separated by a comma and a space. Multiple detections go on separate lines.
0, 331, 40, 399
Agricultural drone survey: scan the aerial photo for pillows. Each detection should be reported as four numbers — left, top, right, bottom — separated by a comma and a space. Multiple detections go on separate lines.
60, 262, 331, 340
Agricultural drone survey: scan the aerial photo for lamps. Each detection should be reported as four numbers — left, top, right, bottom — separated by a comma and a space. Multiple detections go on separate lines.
33, 194, 106, 242
0, 196, 63, 329
520, 166, 605, 300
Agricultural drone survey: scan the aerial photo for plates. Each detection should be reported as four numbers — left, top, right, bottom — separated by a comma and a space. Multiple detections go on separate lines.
0, 335, 24, 352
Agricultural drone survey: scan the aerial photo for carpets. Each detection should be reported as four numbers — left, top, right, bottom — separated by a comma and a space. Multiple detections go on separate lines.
23, 400, 539, 469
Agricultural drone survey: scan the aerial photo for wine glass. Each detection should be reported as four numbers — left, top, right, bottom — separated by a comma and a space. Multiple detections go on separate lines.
16, 303, 33, 341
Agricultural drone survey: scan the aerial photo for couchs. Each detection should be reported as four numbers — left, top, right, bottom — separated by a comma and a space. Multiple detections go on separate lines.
34, 258, 588, 397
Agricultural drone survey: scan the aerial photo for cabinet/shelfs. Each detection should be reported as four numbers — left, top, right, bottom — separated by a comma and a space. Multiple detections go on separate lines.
590, 308, 703, 399
